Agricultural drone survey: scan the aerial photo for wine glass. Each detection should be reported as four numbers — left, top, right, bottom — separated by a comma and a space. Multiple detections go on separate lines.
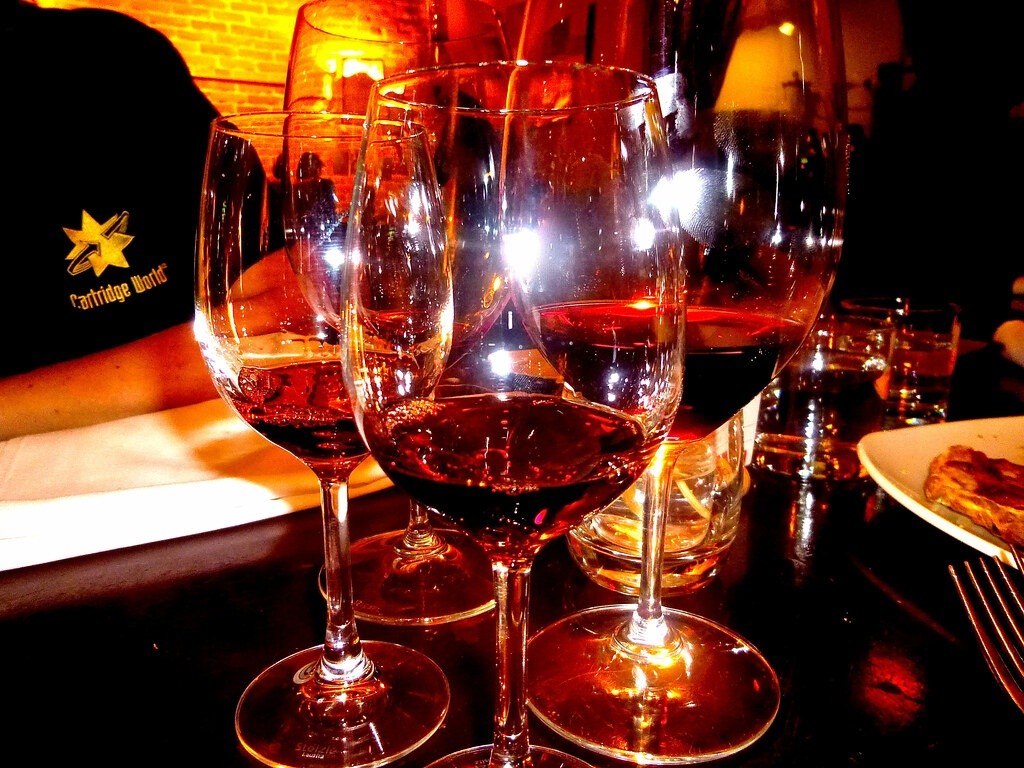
340, 62, 684, 768
498, 0, 850, 765
192, 111, 454, 768
280, 0, 518, 625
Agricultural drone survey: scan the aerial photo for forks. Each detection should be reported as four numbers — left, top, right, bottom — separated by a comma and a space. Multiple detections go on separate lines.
947, 543, 1024, 713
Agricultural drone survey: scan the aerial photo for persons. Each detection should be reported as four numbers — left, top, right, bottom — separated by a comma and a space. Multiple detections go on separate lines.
0, 0, 342, 445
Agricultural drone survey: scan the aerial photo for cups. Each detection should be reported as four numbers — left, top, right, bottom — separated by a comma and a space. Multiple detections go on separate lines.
753, 315, 894, 483
568, 412, 743, 599
840, 296, 960, 429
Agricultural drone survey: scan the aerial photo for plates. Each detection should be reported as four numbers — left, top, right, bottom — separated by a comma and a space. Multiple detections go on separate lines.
856, 416, 1024, 571
